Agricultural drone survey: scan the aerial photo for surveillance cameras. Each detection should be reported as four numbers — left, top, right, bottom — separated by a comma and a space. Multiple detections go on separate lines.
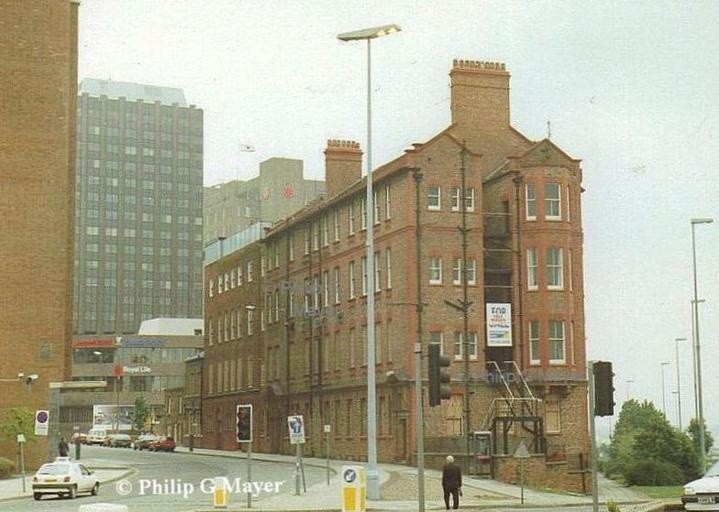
18, 372, 24, 379
27, 374, 38, 381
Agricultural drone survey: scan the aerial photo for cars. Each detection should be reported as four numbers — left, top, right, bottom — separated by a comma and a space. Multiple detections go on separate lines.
31, 456, 100, 500
681, 460, 719, 512
70, 428, 176, 452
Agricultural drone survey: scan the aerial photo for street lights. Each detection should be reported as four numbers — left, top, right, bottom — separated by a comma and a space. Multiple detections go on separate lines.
660, 216, 715, 477
624, 379, 634, 401
336, 22, 405, 502
93, 351, 120, 405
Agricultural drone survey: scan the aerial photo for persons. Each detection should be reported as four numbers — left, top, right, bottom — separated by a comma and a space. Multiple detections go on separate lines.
439, 455, 464, 508
59, 436, 70, 457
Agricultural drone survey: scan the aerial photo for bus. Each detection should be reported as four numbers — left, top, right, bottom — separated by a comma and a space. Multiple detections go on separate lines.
93, 405, 164, 430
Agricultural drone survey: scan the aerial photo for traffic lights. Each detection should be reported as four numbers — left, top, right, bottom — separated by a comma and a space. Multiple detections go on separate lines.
427, 342, 452, 406
236, 404, 253, 442
593, 361, 616, 417
